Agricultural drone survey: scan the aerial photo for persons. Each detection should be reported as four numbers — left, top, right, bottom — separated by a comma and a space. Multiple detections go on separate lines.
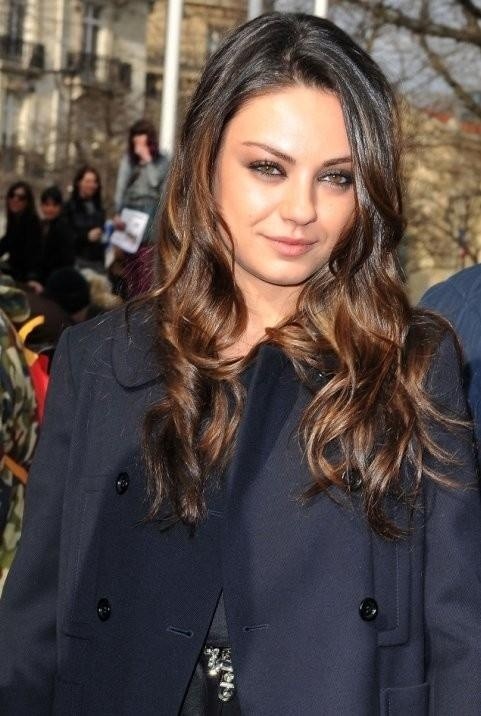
418, 261, 481, 448
0, 118, 172, 595
0, 9, 481, 716
